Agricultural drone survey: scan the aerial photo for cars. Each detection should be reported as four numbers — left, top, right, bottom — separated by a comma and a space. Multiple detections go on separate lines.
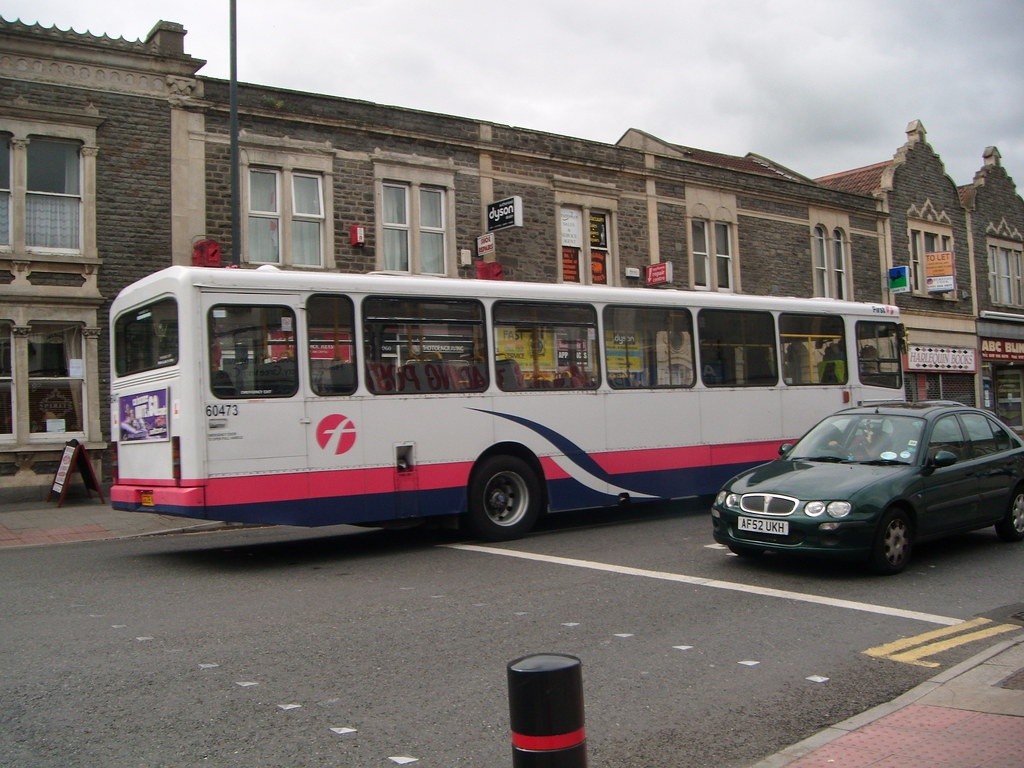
711, 406, 1023, 574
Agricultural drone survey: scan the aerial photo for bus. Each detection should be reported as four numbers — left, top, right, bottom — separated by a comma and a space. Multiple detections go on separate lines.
107, 265, 907, 539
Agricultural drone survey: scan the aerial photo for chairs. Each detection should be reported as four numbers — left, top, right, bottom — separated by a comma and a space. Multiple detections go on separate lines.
214, 343, 870, 400
